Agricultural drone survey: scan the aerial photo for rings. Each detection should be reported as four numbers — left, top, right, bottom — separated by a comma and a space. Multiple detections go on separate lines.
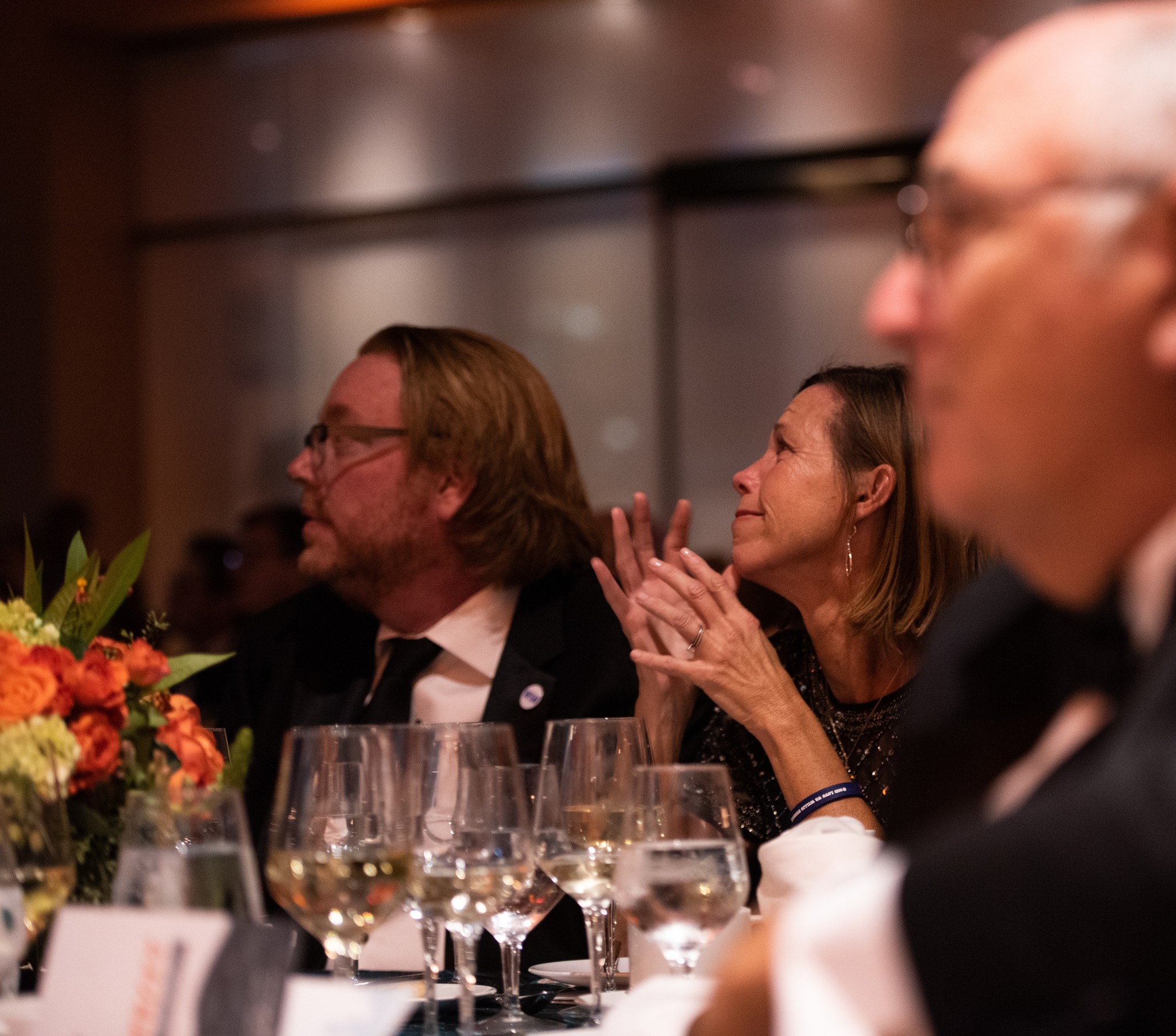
692, 624, 705, 647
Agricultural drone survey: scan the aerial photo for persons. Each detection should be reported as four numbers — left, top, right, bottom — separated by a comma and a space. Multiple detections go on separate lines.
167, 530, 244, 733
206, 501, 382, 974
589, 361, 1000, 911
23, 489, 145, 648
284, 323, 706, 1015
692, 0, 1176, 1036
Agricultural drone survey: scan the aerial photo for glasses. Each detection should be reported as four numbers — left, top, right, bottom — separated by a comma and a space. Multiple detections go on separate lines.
305, 423, 408, 446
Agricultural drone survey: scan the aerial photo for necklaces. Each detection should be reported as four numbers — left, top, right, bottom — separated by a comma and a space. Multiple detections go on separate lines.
819, 650, 908, 769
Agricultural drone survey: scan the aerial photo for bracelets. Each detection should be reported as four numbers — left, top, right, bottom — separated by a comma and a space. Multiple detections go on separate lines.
787, 781, 867, 825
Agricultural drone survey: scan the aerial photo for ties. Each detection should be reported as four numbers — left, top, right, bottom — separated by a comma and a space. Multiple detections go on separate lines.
358, 638, 444, 724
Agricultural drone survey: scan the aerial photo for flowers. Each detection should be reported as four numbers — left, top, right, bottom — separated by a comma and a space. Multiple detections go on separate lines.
1, 501, 253, 979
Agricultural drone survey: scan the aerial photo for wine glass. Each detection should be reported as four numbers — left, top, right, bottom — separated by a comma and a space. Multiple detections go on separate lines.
109, 712, 754, 1035
